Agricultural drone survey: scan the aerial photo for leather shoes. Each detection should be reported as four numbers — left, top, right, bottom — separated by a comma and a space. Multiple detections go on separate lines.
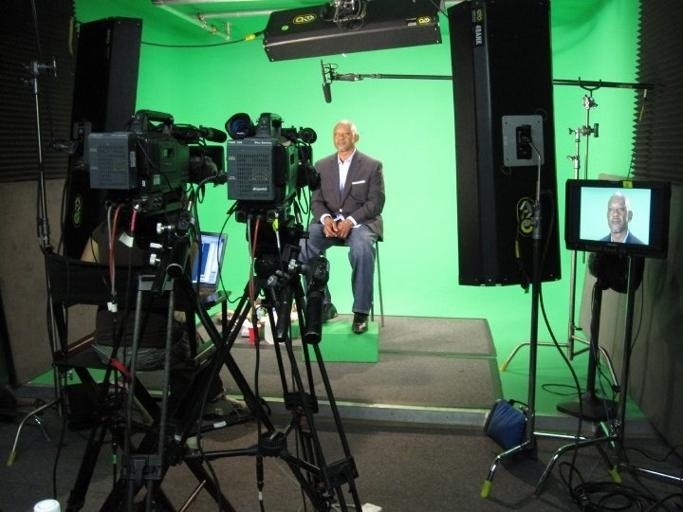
352, 314, 368, 334
320, 302, 338, 321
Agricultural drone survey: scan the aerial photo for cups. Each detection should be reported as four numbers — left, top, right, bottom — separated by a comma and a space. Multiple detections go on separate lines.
247, 324, 262, 345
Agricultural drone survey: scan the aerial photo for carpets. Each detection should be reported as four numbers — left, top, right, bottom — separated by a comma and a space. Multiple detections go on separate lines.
0, 383, 682, 512
122, 347, 497, 411
192, 310, 491, 356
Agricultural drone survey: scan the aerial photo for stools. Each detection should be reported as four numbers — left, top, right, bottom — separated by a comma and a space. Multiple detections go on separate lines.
323, 233, 382, 323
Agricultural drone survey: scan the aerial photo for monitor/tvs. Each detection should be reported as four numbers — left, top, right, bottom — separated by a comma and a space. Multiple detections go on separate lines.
192, 232, 227, 287
565, 179, 671, 260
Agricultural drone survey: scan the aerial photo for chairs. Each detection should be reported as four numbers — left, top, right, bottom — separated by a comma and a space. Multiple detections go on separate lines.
41, 246, 194, 416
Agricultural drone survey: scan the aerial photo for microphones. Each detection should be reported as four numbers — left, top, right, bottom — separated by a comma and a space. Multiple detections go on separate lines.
321, 59, 332, 103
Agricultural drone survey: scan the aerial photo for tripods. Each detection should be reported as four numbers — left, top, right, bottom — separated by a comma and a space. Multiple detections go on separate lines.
65, 198, 334, 512
480, 217, 622, 499
536, 258, 683, 497
6, 76, 151, 467
101, 215, 362, 512
499, 96, 622, 399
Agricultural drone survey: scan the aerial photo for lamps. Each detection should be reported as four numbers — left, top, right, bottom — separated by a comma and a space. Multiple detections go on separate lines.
254, 0, 448, 63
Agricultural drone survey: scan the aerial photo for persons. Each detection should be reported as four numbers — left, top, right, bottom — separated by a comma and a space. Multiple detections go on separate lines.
81, 208, 234, 413
295, 118, 385, 337
595, 191, 644, 246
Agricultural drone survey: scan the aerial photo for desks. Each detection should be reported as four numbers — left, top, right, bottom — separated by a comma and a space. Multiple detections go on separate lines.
189, 290, 230, 363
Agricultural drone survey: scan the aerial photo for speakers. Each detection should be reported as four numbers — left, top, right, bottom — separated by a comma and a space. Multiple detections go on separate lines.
61, 16, 143, 262
447, 0, 561, 287
